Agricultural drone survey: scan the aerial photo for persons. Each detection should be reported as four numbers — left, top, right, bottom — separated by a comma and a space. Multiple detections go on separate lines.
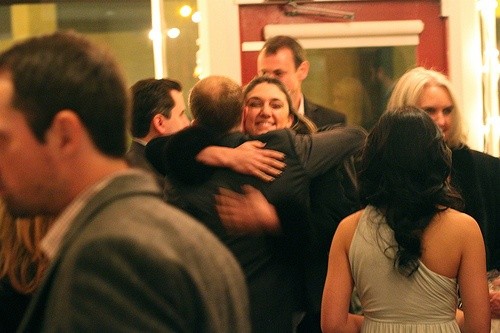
145, 75, 369, 333
255, 35, 361, 333
320, 105, 491, 333
160, 75, 370, 333
128, 79, 191, 166
0, 31, 253, 333
385, 67, 500, 333
